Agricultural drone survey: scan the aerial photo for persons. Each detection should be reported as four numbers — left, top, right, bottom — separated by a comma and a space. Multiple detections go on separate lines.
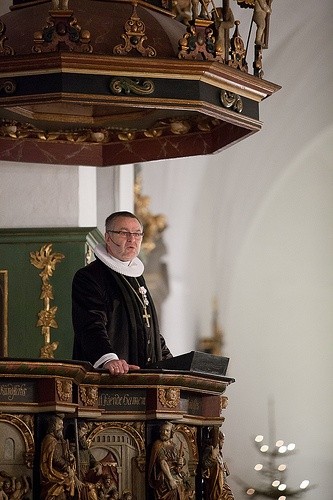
0, 475, 29, 500
148, 421, 194, 500
201, 428, 234, 500
72, 212, 175, 376
39, 416, 119, 500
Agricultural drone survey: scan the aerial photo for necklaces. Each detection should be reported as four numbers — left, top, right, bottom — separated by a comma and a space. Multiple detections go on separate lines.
121, 274, 150, 328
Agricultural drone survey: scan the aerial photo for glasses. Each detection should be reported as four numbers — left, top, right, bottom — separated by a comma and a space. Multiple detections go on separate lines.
106, 230, 144, 239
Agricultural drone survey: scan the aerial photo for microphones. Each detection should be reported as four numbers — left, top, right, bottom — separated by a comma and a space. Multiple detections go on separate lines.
108, 232, 120, 246
